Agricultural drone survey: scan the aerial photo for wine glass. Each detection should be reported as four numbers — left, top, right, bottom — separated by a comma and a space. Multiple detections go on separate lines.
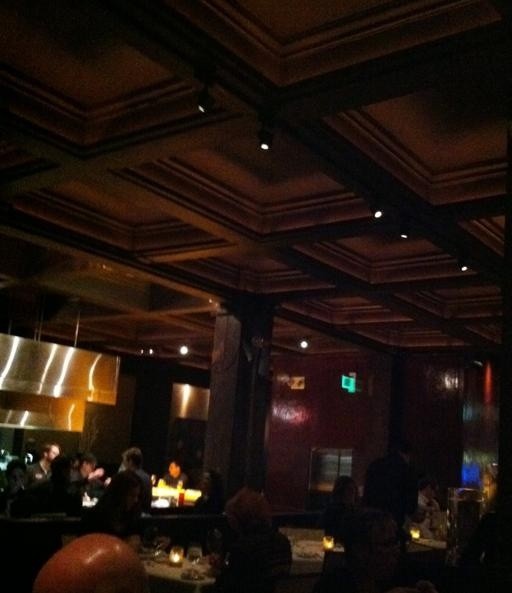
186, 547, 203, 581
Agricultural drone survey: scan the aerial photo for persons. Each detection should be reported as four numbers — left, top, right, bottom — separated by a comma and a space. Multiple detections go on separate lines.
363, 443, 418, 526
417, 479, 440, 512
460, 495, 512, 593
194, 471, 227, 514
162, 461, 188, 488
312, 508, 436, 592
321, 477, 362, 533
211, 488, 292, 591
0, 437, 152, 541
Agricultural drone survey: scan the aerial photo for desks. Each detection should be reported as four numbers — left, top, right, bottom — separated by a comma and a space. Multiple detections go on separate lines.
141, 554, 216, 592
